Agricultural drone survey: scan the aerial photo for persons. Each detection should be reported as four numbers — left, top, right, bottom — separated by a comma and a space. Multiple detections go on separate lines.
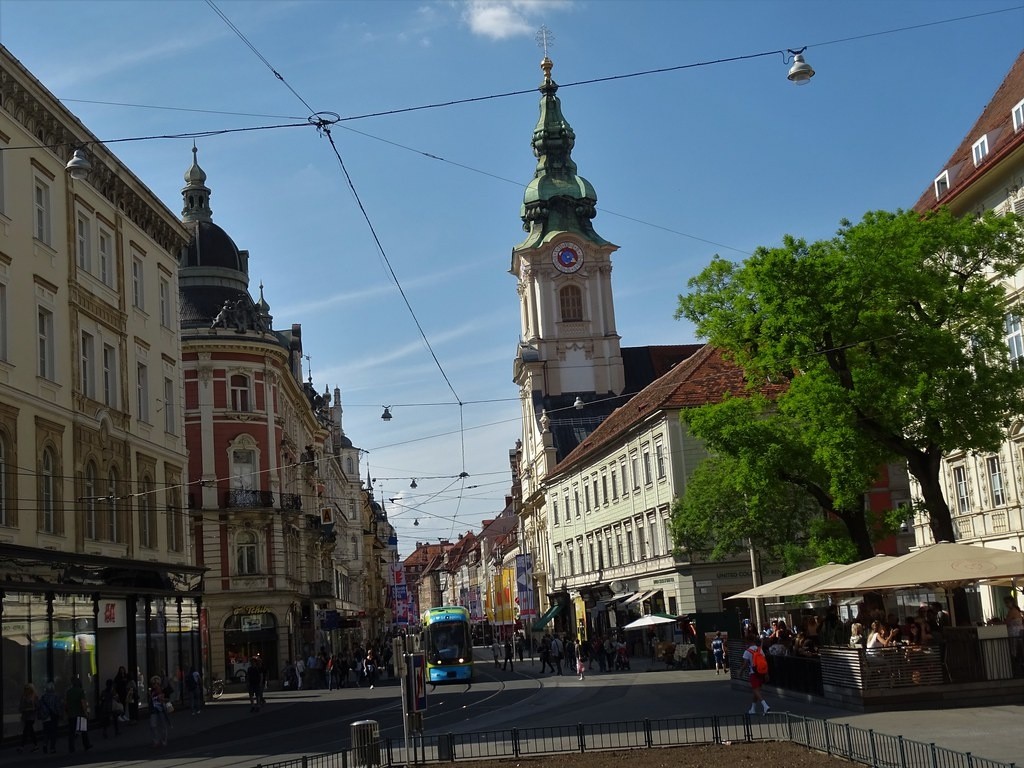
738, 635, 770, 716
19, 682, 60, 754
710, 631, 729, 675
65, 675, 93, 753
491, 631, 629, 681
352, 650, 364, 686
147, 675, 169, 747
294, 646, 354, 691
744, 596, 1024, 692
187, 667, 203, 715
365, 625, 405, 670
248, 658, 266, 712
363, 649, 377, 690
99, 666, 129, 739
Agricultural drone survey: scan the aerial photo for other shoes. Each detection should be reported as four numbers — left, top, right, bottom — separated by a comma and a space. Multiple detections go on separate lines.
17, 745, 95, 753
494, 663, 612, 681
250, 707, 255, 712
192, 712, 195, 715
197, 711, 200, 714
725, 670, 728, 673
369, 685, 374, 690
115, 731, 121, 736
763, 707, 770, 716
151, 740, 159, 748
716, 672, 719, 675
103, 734, 108, 740
748, 710, 755, 714
255, 706, 260, 712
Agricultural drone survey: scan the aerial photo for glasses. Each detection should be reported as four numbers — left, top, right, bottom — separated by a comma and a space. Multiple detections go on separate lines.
911, 626, 915, 629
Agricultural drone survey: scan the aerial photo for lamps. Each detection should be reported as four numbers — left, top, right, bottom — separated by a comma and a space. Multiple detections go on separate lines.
64, 148, 94, 182
413, 518, 419, 526
381, 407, 393, 422
786, 46, 816, 85
410, 479, 417, 489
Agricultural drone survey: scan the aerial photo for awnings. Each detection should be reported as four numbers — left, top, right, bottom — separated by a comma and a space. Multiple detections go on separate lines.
590, 588, 663, 617
531, 605, 564, 632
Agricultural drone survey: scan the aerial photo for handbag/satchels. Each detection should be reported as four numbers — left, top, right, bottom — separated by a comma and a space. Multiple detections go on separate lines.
558, 652, 564, 659
111, 697, 124, 715
579, 654, 587, 662
300, 671, 305, 677
165, 701, 174, 714
76, 712, 88, 732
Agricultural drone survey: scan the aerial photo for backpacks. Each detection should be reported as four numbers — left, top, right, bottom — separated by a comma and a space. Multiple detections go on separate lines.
185, 672, 199, 691
747, 646, 768, 675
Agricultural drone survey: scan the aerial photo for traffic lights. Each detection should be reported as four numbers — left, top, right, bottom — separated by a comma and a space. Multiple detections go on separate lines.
400, 629, 406, 640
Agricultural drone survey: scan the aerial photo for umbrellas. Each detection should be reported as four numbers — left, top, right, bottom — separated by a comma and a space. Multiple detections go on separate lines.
723, 540, 1024, 600
621, 613, 677, 664
31, 630, 95, 653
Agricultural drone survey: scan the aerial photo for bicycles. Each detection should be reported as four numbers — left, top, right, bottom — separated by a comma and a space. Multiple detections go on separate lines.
212, 677, 224, 699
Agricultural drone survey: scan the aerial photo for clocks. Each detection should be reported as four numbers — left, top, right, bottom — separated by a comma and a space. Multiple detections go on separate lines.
552, 242, 584, 273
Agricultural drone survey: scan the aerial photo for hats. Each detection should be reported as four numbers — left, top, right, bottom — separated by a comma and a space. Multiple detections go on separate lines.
150, 676, 161, 682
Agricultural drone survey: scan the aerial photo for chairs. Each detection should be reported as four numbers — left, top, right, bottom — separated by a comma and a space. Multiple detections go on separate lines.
861, 651, 893, 690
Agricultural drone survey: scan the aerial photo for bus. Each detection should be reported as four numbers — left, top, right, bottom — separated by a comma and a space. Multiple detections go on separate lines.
422, 606, 476, 684
20, 627, 201, 711
